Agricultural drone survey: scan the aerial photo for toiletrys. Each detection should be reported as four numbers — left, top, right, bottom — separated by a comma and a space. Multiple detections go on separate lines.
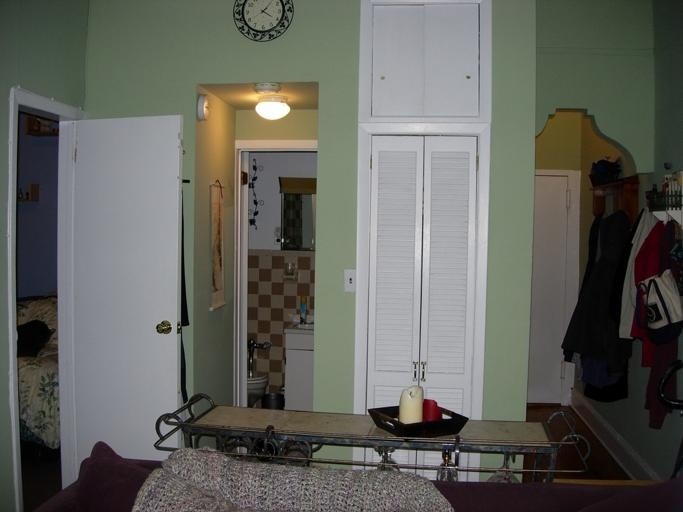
300, 303, 307, 324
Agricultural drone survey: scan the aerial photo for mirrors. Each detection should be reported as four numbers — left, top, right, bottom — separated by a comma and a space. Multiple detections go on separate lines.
281, 193, 314, 251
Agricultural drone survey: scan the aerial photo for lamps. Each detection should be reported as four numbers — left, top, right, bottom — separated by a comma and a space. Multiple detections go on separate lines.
255, 95, 291, 120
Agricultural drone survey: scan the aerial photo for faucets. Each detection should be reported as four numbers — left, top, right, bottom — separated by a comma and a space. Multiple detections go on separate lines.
247, 338, 255, 378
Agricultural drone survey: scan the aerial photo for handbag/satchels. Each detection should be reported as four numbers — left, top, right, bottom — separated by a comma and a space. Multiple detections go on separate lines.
638, 269, 683, 344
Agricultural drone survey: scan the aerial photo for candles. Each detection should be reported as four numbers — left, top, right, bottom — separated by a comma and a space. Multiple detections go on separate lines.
399, 385, 441, 424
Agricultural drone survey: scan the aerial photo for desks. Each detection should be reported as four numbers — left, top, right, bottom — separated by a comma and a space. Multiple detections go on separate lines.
154, 392, 591, 481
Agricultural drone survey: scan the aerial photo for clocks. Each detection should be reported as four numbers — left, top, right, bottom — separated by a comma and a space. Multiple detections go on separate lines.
232, 1, 295, 42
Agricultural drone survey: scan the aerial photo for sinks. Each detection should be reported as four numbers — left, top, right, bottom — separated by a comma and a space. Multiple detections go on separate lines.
247, 372, 268, 397
